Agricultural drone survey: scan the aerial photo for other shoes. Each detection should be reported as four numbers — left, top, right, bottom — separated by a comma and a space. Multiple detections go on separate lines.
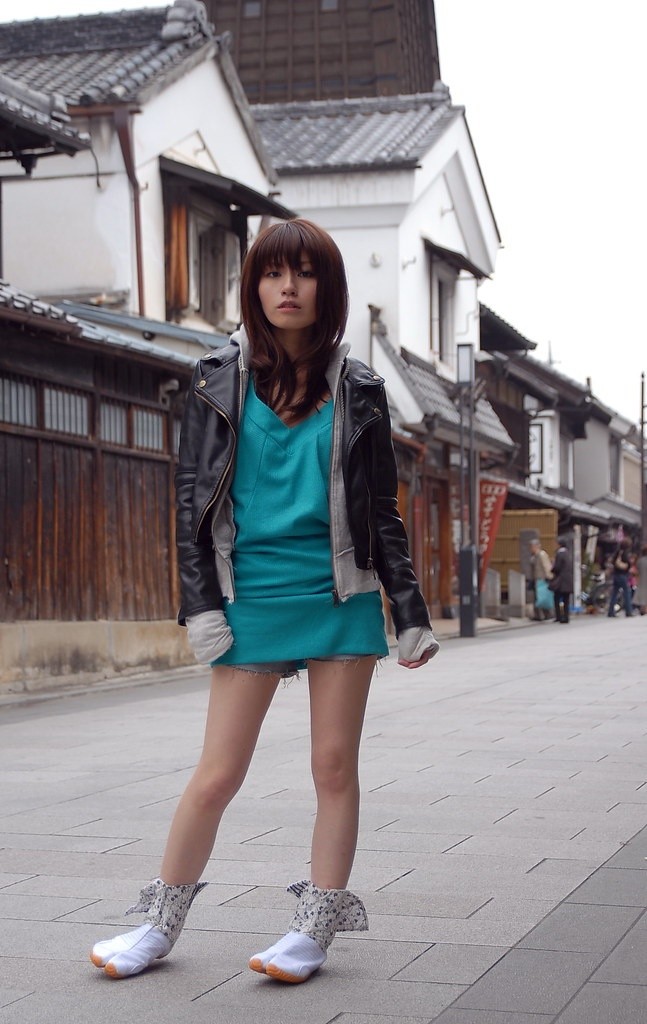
249, 880, 369, 983
91, 877, 209, 984
529, 615, 544, 621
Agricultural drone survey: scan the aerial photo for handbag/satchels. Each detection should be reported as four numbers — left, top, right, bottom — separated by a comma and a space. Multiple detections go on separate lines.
534, 578, 555, 609
614, 550, 629, 570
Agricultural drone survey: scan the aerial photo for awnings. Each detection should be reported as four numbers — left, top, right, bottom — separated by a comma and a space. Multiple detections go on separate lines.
159, 155, 298, 219
423, 237, 493, 280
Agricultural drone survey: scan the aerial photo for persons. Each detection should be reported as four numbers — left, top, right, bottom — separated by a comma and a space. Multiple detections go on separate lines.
92, 214, 439, 985
528, 535, 647, 623
